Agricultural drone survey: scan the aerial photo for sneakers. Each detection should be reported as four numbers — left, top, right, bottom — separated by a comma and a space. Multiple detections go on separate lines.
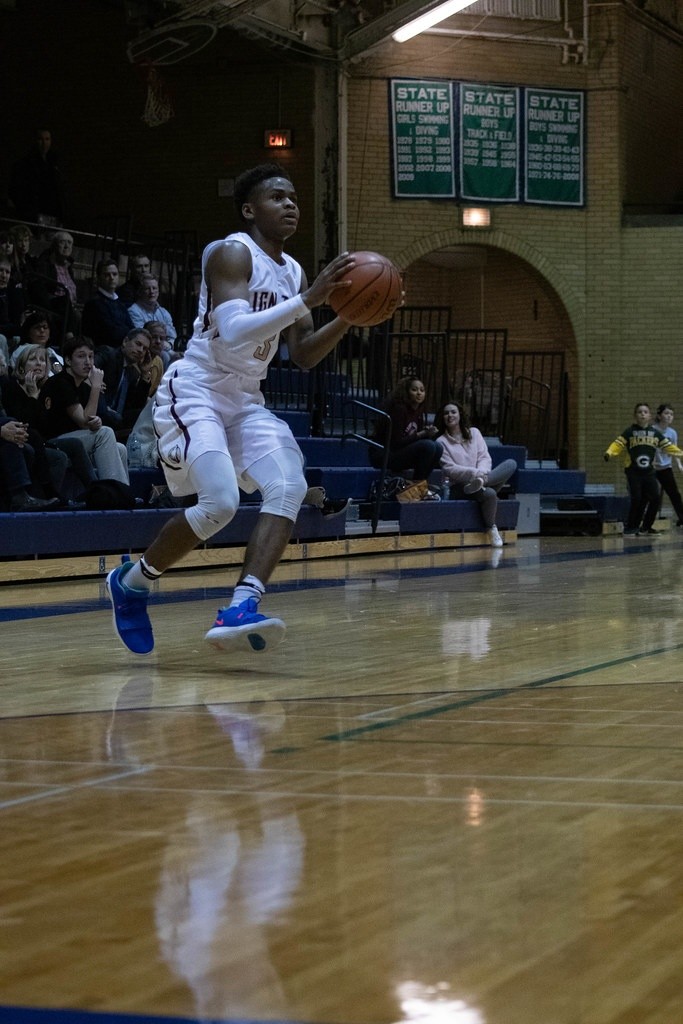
303, 486, 326, 505
205, 596, 286, 654
487, 524, 504, 548
623, 526, 662, 538
105, 561, 155, 656
317, 497, 354, 520
8, 493, 60, 512
464, 477, 486, 494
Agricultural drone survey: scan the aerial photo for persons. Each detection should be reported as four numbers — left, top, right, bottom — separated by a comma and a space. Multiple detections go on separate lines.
104, 161, 405, 656
434, 401, 517, 548
604, 403, 683, 536
1, 224, 353, 520
368, 377, 443, 501
39, 335, 129, 510
650, 403, 683, 525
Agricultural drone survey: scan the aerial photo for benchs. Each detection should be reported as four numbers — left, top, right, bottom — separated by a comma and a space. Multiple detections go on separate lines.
488, 446, 528, 470
264, 368, 351, 395
0, 503, 347, 560
556, 494, 629, 521
271, 409, 312, 437
294, 437, 370, 467
323, 392, 381, 418
357, 501, 520, 535
94, 469, 262, 503
509, 469, 587, 495
303, 467, 444, 500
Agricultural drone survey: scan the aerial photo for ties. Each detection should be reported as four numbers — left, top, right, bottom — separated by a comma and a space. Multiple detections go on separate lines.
115, 367, 128, 415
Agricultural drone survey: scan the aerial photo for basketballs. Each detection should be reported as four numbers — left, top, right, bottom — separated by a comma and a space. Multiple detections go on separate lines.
330, 251, 403, 327
